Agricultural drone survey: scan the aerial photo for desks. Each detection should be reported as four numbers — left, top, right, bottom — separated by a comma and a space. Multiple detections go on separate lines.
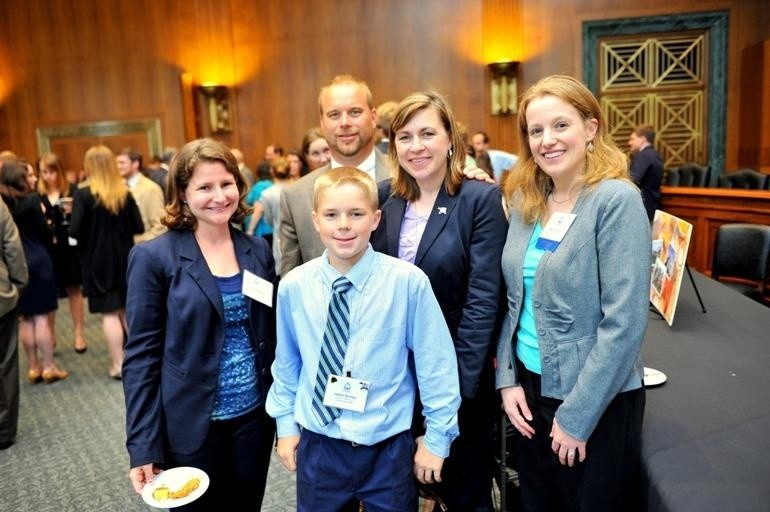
651, 184, 770, 299
634, 265, 770, 512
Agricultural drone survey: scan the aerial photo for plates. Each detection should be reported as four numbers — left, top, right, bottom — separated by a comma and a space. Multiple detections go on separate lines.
642, 365, 667, 388
141, 467, 211, 509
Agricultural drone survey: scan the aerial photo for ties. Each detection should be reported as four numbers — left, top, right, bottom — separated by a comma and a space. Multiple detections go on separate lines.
310, 276, 355, 428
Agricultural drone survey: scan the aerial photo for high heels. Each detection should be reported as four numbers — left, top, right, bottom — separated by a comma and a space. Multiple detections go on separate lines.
40, 368, 68, 385
26, 369, 42, 386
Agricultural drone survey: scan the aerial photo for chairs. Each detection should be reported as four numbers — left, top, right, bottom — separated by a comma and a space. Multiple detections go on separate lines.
707, 219, 770, 300
664, 159, 713, 190
717, 167, 768, 188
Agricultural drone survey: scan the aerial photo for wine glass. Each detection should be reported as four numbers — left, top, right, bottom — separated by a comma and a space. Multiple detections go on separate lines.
58, 199, 72, 226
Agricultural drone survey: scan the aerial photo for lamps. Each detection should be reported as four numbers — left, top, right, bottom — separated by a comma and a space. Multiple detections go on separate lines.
199, 81, 237, 138
486, 59, 521, 118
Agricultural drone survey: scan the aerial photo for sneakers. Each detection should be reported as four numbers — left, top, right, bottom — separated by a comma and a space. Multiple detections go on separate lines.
72, 336, 87, 353
107, 366, 122, 379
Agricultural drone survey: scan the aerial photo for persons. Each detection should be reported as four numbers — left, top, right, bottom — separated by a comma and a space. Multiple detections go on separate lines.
459, 127, 521, 188
264, 167, 462, 512
372, 100, 399, 156
122, 137, 280, 512
229, 128, 332, 253
367, 89, 510, 511
493, 74, 654, 512
628, 122, 660, 219
280, 74, 394, 283
